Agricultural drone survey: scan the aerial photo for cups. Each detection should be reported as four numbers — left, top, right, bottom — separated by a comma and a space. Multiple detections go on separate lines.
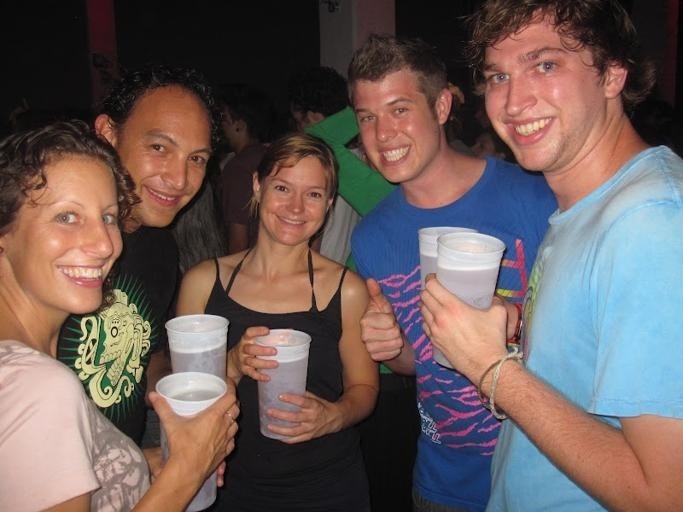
256, 328, 311, 441
155, 372, 228, 512
165, 313, 231, 383
418, 226, 507, 371
